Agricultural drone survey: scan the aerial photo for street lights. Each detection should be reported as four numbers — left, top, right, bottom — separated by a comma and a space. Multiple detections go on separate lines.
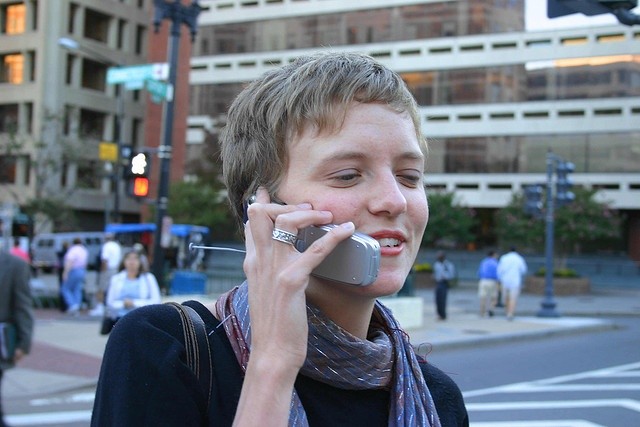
58, 38, 125, 221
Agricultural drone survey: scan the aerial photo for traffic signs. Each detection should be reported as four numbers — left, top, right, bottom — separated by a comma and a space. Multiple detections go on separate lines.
147, 81, 174, 102
106, 62, 170, 91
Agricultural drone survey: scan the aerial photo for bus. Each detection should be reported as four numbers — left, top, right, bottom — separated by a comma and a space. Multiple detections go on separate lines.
31, 232, 104, 273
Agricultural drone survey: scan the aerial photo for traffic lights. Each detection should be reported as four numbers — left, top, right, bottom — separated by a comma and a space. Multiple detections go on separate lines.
556, 161, 576, 205
525, 185, 544, 215
128, 176, 150, 197
126, 152, 150, 175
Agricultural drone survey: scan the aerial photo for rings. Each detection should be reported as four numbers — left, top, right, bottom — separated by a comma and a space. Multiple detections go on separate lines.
271, 227, 299, 246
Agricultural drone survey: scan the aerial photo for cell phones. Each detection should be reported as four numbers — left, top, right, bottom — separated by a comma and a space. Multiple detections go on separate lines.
243, 195, 381, 286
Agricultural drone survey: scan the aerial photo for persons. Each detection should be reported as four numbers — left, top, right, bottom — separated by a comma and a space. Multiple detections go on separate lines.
8, 239, 31, 264
132, 243, 149, 271
105, 251, 163, 324
497, 246, 529, 320
432, 254, 453, 319
90, 45, 470, 427
97, 232, 122, 309
60, 239, 90, 315
0, 219, 36, 427
477, 250, 502, 318
57, 241, 70, 312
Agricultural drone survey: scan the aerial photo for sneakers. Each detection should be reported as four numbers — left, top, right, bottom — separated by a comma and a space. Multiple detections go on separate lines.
89, 305, 104, 317
64, 303, 79, 315
